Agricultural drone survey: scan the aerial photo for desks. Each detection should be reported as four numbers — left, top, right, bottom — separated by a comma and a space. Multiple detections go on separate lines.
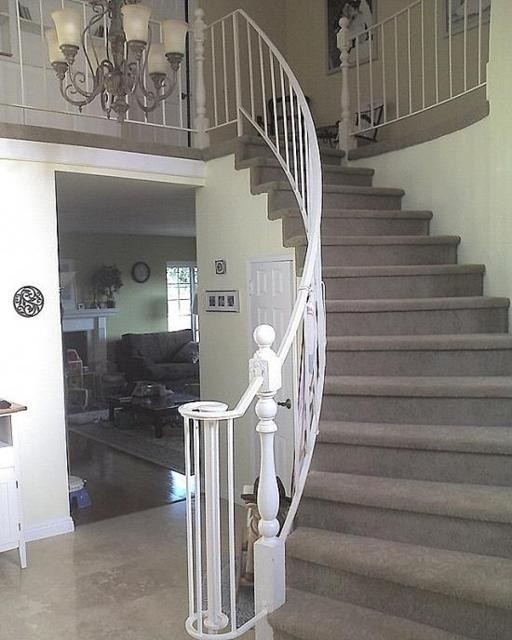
315, 124, 339, 147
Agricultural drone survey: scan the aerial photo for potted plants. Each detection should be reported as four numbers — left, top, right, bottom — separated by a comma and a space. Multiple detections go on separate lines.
94, 265, 125, 307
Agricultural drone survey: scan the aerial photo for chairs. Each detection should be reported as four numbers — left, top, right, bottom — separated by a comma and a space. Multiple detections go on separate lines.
255, 95, 311, 146
352, 104, 384, 143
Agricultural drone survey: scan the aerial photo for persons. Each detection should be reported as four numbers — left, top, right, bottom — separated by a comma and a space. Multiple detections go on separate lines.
332, 0, 372, 67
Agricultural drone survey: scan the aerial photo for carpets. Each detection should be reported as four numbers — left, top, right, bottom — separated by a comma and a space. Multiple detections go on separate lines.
68, 422, 201, 476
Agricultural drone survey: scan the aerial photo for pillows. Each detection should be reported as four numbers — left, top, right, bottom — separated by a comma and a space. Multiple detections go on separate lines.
172, 339, 200, 364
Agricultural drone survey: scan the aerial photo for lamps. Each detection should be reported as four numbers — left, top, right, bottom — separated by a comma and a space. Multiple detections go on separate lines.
48, 0, 189, 124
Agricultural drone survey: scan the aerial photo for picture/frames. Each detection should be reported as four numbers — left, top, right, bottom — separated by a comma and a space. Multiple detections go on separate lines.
326, 1, 379, 75
206, 290, 240, 312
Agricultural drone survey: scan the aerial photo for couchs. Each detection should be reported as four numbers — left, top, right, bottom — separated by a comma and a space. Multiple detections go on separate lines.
106, 330, 201, 382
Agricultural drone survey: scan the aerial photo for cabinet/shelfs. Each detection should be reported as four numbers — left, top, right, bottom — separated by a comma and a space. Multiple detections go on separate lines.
0, 414, 27, 570
